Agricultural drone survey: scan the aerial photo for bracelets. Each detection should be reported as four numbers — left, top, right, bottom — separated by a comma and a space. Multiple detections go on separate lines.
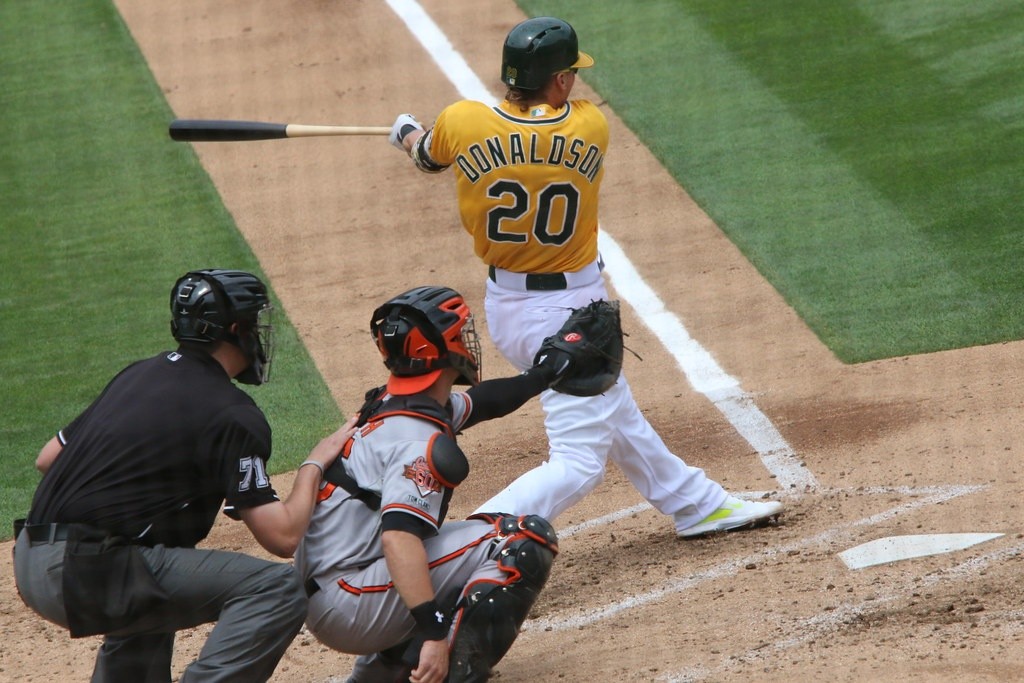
410, 599, 450, 641
298, 461, 324, 476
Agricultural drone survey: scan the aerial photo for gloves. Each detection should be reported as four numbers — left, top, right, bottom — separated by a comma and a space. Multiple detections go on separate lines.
388, 113, 423, 151
527, 348, 573, 390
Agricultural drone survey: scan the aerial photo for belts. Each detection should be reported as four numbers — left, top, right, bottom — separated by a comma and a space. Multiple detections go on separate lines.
24, 521, 69, 542
304, 565, 364, 598
490, 252, 605, 291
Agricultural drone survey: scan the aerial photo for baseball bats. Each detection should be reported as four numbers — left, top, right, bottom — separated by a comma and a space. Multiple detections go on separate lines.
168, 117, 428, 143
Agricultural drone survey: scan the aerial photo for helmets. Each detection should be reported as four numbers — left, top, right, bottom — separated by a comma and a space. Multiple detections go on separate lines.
373, 306, 450, 395
170, 268, 273, 387
501, 17, 594, 90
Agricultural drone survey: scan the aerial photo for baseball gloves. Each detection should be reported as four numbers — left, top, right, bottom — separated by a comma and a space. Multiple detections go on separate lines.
532, 299, 625, 397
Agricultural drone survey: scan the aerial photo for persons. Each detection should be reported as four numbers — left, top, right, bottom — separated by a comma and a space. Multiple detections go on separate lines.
390, 18, 785, 538
11, 268, 357, 683
295, 284, 624, 683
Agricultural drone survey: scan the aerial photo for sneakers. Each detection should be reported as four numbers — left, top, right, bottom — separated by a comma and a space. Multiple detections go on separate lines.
676, 494, 785, 537
